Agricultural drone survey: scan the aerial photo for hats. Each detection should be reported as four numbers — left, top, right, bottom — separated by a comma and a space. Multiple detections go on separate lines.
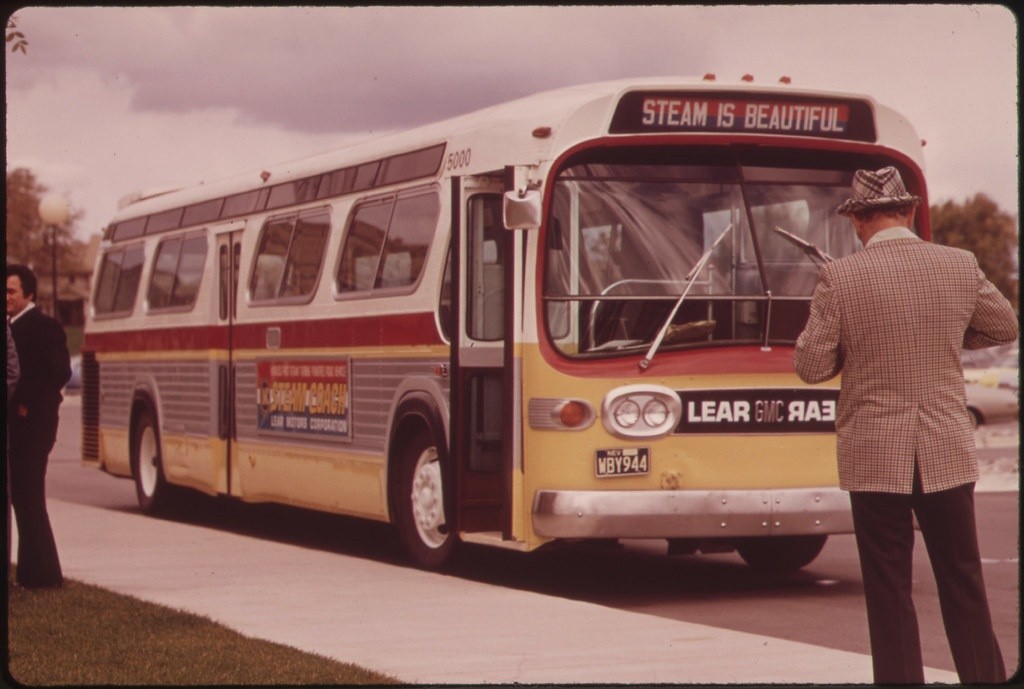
836, 166, 922, 216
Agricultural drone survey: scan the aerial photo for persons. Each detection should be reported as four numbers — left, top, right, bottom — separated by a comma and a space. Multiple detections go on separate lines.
792, 169, 1019, 684
5, 264, 73, 588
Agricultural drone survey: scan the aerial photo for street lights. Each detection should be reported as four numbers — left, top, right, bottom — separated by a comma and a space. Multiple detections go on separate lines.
36, 191, 70, 324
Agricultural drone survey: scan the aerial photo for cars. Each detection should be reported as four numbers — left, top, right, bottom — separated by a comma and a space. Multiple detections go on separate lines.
960, 342, 1020, 430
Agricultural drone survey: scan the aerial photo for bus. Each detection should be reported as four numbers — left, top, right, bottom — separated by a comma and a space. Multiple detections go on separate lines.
78, 75, 933, 574
